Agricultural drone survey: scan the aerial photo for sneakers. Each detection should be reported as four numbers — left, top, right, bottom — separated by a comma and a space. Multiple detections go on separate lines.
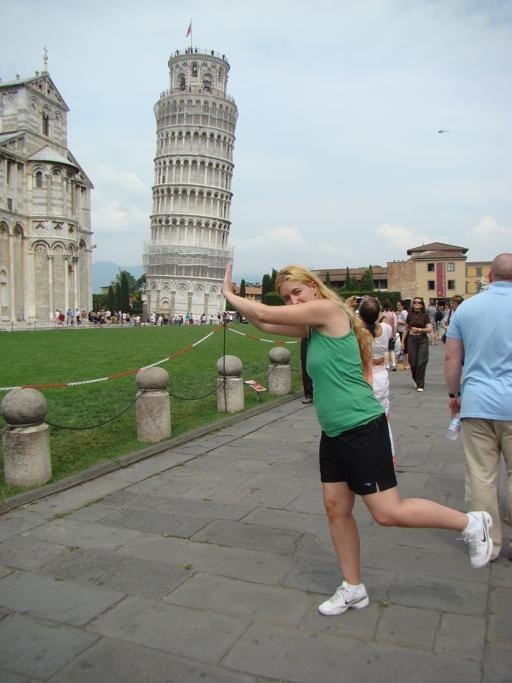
318, 580, 370, 616
455, 511, 492, 567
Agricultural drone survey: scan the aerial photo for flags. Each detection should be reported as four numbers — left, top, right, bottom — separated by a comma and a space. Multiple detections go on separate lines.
186, 24, 191, 37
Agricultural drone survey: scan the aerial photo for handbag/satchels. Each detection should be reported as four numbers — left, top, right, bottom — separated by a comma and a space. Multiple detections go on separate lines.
443, 334, 447, 343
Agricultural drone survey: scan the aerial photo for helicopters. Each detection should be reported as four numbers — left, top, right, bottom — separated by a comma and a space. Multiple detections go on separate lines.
438, 128, 450, 133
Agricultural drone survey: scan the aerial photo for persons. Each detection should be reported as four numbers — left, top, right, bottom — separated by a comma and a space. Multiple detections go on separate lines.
444, 255, 512, 561
301, 292, 465, 467
222, 258, 492, 616
54, 306, 228, 327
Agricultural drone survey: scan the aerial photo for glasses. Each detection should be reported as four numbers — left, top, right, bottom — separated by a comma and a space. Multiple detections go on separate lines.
413, 301, 422, 304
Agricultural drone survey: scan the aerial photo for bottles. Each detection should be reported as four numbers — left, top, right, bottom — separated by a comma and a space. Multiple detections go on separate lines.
447, 412, 462, 438
